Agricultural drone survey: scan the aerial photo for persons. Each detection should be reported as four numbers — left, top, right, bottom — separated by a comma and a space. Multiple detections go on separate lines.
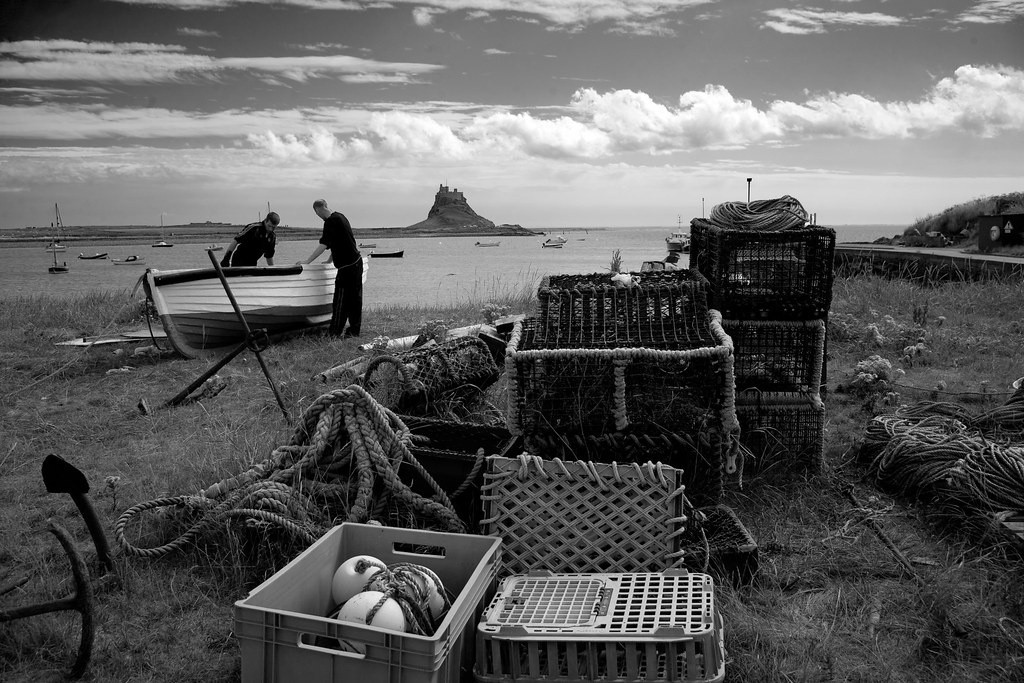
295, 199, 362, 338
219, 212, 280, 267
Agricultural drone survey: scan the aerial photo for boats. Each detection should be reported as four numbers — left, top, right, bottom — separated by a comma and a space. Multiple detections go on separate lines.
205, 246, 223, 251
665, 213, 690, 254
78, 251, 110, 260
358, 244, 376, 248
368, 250, 404, 258
48, 222, 69, 273
152, 214, 173, 248
45, 202, 67, 253
542, 242, 563, 249
111, 255, 147, 265
144, 256, 369, 361
474, 242, 500, 246
547, 236, 568, 243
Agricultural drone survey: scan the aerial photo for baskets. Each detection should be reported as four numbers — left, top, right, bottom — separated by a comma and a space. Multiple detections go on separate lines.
503, 208, 836, 583
365, 335, 499, 416
475, 569, 727, 683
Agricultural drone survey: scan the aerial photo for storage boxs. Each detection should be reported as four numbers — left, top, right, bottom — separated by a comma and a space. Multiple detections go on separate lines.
229, 216, 839, 683
